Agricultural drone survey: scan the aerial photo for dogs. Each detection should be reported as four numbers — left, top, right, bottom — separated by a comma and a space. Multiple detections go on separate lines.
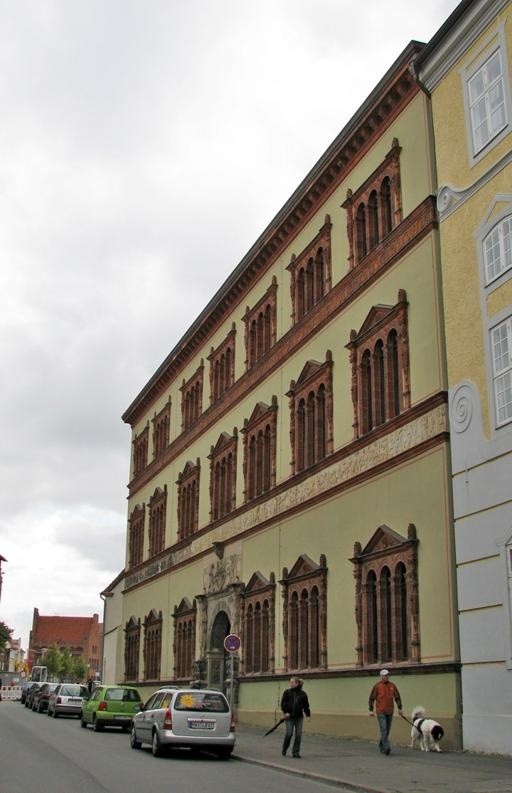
410, 706, 444, 752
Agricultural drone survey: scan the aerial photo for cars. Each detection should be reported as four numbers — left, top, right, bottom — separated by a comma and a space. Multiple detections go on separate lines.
22, 680, 101, 717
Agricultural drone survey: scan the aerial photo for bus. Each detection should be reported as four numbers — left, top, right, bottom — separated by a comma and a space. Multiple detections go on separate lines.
26, 664, 78, 692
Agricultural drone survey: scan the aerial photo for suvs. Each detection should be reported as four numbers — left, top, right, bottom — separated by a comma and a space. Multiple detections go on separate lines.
81, 684, 145, 733
129, 685, 236, 761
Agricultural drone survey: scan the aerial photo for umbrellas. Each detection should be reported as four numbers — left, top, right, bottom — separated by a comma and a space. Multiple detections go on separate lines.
263, 714, 285, 739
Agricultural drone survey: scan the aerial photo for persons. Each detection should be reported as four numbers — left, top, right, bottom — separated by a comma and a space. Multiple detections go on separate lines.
367, 669, 402, 757
280, 676, 313, 759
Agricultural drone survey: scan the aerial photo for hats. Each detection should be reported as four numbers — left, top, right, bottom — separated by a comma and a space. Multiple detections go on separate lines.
380, 669, 390, 675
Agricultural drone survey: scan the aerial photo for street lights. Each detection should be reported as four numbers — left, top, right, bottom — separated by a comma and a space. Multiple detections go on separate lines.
194, 655, 208, 689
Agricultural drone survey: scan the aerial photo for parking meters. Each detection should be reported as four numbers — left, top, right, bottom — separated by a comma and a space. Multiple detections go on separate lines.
87, 677, 93, 693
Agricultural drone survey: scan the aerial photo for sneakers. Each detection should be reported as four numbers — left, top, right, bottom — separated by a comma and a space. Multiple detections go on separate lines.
282, 751, 285, 755
378, 743, 391, 755
292, 751, 301, 758
